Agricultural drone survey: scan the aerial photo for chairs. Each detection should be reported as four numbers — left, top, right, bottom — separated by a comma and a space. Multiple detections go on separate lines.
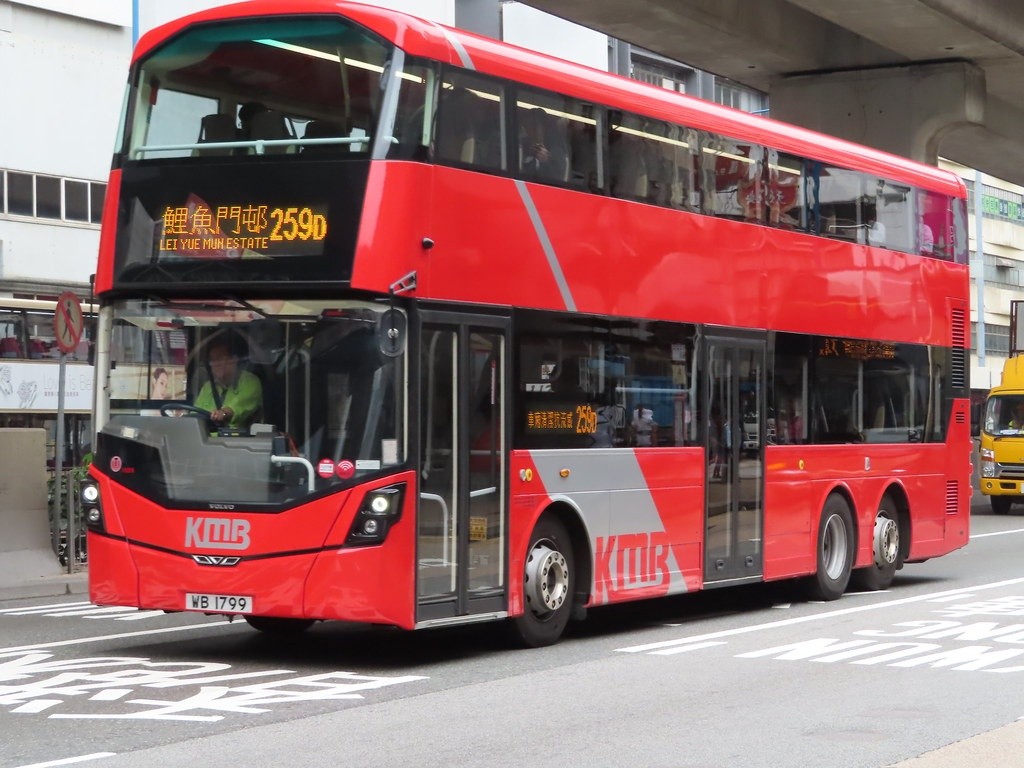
190, 112, 350, 157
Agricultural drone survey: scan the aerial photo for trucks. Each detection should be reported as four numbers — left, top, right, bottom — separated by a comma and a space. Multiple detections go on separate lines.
971, 299, 1024, 514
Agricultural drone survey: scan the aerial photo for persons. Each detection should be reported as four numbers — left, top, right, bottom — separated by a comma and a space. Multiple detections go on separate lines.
140, 366, 175, 418
187, 337, 264, 438
521, 106, 562, 182
863, 206, 935, 255
590, 390, 728, 480
1007, 404, 1024, 431
232, 101, 270, 156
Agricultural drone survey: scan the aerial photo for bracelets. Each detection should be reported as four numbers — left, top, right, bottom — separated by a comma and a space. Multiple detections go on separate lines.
219, 408, 229, 420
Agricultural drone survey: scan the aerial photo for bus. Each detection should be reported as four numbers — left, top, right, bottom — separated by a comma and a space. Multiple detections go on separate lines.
80, 0, 974, 652
